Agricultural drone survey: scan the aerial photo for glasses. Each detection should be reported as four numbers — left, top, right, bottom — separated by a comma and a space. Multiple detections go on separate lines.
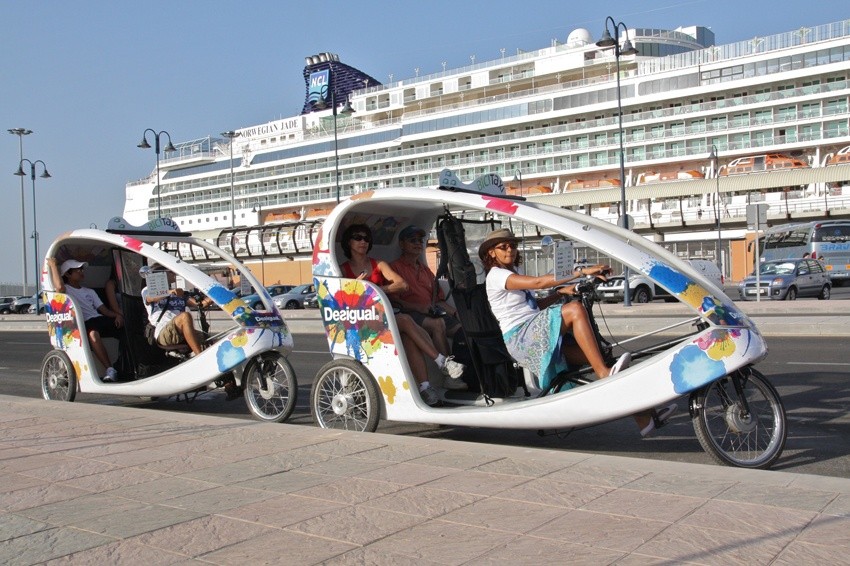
350, 234, 371, 243
71, 269, 85, 273
822, 259, 824, 260
408, 239, 423, 243
495, 243, 516, 251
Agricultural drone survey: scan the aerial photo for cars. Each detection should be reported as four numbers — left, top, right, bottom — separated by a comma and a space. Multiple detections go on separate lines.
740, 258, 832, 302
302, 291, 319, 309
239, 284, 298, 310
0, 289, 45, 315
270, 283, 314, 310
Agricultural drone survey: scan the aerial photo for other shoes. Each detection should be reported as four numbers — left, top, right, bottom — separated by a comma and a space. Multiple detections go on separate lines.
224, 387, 243, 400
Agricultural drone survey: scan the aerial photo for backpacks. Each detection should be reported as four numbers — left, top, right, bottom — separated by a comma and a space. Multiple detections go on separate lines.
433, 214, 478, 312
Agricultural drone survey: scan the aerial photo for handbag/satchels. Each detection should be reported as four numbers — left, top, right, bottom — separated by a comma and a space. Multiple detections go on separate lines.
144, 323, 155, 345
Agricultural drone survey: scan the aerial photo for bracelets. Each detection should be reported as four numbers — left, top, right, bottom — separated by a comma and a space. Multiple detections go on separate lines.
577, 267, 586, 278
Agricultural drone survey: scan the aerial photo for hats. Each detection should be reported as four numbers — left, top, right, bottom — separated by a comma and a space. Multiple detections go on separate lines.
150, 263, 172, 273
478, 228, 522, 260
399, 225, 426, 238
60, 259, 88, 276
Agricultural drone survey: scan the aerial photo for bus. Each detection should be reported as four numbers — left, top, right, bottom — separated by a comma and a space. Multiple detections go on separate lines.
747, 219, 850, 287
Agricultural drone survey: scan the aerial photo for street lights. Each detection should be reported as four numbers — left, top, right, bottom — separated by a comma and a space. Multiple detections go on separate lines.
596, 15, 640, 306
6, 128, 35, 296
221, 130, 242, 228
14, 158, 54, 316
137, 128, 179, 218
312, 85, 358, 203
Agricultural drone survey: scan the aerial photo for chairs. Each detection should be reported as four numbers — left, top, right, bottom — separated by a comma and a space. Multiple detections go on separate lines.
113, 247, 190, 380
436, 215, 530, 403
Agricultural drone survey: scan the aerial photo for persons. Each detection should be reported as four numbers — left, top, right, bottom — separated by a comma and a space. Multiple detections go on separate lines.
45, 257, 126, 383
103, 261, 124, 318
477, 228, 631, 394
141, 261, 217, 355
383, 225, 468, 387
340, 224, 466, 405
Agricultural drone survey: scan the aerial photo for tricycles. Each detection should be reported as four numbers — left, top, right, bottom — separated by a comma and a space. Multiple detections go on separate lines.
39, 211, 299, 423
307, 167, 790, 472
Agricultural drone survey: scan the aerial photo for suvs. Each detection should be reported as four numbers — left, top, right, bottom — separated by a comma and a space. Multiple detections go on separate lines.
595, 254, 726, 303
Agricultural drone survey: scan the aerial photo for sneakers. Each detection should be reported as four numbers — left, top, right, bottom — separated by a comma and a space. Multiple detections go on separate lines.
439, 355, 464, 380
443, 377, 467, 389
103, 367, 117, 382
420, 386, 438, 405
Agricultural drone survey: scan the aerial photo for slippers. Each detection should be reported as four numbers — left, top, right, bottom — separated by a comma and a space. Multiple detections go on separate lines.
639, 403, 677, 436
610, 352, 631, 376
